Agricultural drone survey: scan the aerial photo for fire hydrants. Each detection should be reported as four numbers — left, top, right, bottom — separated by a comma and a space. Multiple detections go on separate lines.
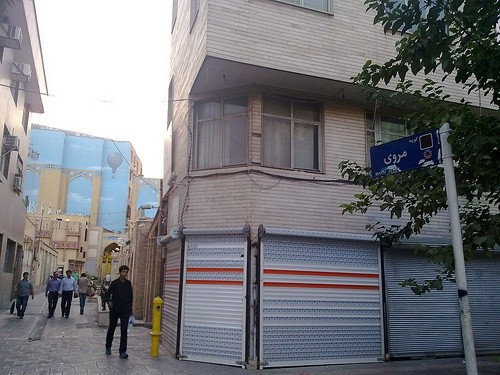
148, 295, 164, 358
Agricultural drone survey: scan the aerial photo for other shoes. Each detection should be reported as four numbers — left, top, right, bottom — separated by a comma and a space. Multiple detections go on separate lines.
48, 314, 54, 318
17, 313, 20, 316
20, 315, 23, 318
106, 347, 111, 354
62, 313, 69, 318
120, 352, 128, 358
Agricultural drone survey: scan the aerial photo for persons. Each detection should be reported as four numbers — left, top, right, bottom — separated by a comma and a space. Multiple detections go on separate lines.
72, 270, 79, 282
76, 273, 92, 315
12, 272, 34, 319
58, 269, 77, 318
45, 271, 61, 319
105, 265, 133, 359
105, 273, 111, 286
